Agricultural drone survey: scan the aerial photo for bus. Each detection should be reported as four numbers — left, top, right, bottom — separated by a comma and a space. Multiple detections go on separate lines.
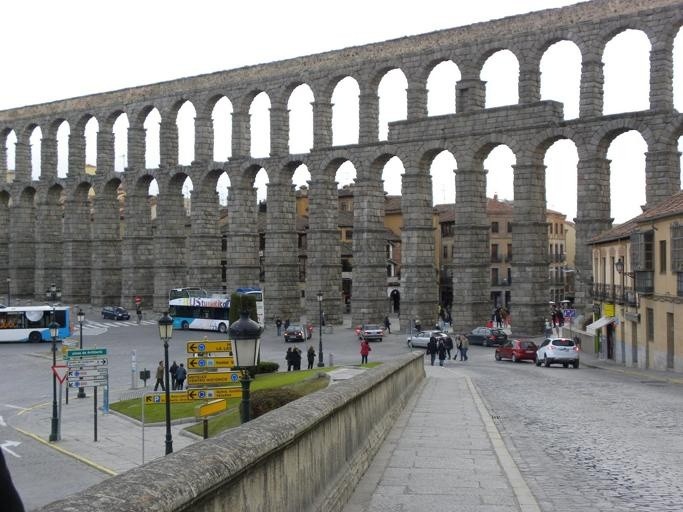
0, 304, 73, 343
167, 286, 266, 337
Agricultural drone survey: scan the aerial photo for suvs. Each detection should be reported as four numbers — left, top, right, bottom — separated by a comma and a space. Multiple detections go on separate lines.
534, 337, 579, 369
101, 305, 130, 319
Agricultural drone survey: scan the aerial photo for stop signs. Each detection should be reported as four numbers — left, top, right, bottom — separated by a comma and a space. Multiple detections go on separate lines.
134, 296, 141, 305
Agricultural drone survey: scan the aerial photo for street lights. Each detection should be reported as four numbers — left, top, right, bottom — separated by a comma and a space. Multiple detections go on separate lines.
74, 310, 87, 397
315, 290, 325, 367
229, 294, 262, 424
45, 281, 61, 303
5, 276, 15, 307
44, 310, 64, 436
157, 310, 174, 454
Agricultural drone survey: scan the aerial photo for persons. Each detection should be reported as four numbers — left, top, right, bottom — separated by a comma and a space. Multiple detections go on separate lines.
429, 334, 469, 366
176, 364, 186, 390
276, 317, 282, 336
360, 340, 369, 363
170, 361, 179, 390
154, 361, 165, 391
495, 304, 512, 328
322, 309, 327, 326
284, 320, 290, 329
384, 316, 392, 334
285, 346, 316, 371
551, 305, 564, 329
544, 319, 552, 338
136, 306, 143, 324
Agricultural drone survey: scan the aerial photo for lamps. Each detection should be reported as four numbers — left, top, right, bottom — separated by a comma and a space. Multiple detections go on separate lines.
615, 258, 635, 279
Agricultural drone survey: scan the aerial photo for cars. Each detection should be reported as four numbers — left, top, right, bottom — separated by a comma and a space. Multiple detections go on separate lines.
354, 325, 361, 336
466, 325, 506, 347
283, 324, 313, 342
406, 329, 449, 349
497, 338, 541, 364
359, 323, 383, 341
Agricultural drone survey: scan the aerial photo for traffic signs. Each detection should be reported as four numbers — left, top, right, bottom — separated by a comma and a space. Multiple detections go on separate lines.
186, 340, 234, 352
187, 386, 242, 399
67, 360, 109, 368
144, 391, 195, 406
66, 370, 108, 378
68, 381, 109, 389
67, 348, 108, 357
186, 356, 236, 365
187, 372, 244, 384
